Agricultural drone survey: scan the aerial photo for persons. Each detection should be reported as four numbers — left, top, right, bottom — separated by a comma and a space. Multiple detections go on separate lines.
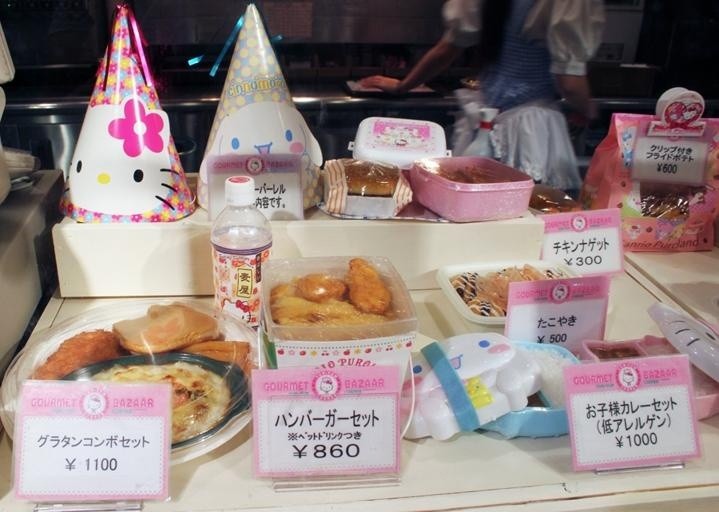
359, 0, 610, 201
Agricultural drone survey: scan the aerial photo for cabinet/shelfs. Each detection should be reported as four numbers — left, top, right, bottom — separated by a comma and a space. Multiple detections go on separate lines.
462, 108, 500, 158
207, 175, 274, 366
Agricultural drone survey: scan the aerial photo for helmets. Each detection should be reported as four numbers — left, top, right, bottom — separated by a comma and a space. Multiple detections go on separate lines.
54, 353, 249, 449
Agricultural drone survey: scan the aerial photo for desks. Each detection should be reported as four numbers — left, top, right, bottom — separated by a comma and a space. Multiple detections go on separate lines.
625, 250, 718, 333
0, 173, 719, 512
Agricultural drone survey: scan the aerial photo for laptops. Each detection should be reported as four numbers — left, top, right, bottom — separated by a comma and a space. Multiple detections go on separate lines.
438, 164, 512, 182
112, 303, 219, 354
177, 341, 252, 373
450, 263, 570, 317
641, 192, 689, 218
270, 257, 394, 342
28, 329, 121, 381
344, 165, 400, 197
530, 184, 583, 213
